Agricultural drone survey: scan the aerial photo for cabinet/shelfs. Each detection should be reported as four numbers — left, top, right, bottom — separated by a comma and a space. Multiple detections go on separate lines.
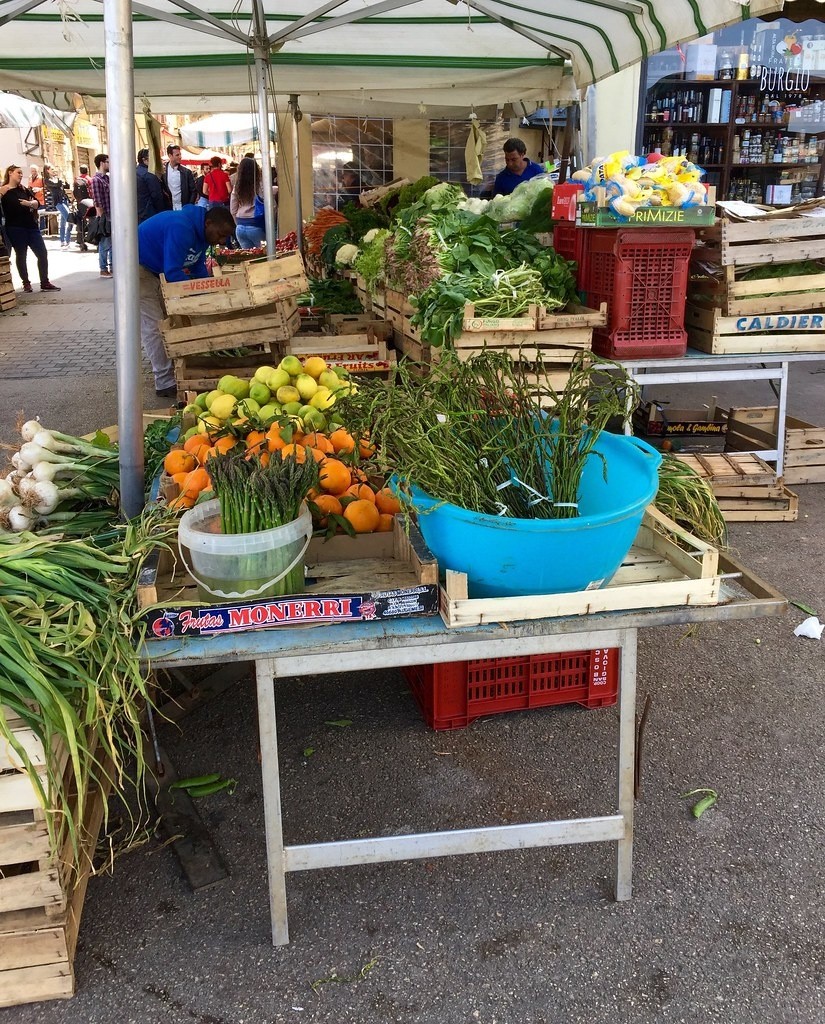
639, 50, 824, 208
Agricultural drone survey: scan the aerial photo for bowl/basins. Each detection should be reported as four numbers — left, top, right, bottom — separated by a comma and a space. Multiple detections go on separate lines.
390, 417, 662, 599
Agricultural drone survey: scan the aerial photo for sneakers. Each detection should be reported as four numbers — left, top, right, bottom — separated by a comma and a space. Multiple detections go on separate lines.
23, 283, 33, 292
40, 282, 61, 291
100, 270, 113, 278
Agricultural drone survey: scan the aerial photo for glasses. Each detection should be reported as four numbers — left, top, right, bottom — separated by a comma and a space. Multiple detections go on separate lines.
169, 145, 176, 153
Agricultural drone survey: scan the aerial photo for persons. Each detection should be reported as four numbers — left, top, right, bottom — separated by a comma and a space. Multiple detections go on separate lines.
137, 204, 236, 398
546, 153, 560, 172
0, 155, 110, 293
313, 138, 393, 211
491, 138, 547, 234
135, 144, 280, 249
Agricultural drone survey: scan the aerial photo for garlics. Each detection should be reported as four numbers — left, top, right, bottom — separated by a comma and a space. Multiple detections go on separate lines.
0, 416, 61, 533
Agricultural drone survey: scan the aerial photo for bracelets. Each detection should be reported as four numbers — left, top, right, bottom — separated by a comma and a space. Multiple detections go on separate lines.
0, 192, 4, 196
29, 201, 33, 208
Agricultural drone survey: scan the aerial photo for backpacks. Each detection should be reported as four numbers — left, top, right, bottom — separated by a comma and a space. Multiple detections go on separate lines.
73, 175, 90, 200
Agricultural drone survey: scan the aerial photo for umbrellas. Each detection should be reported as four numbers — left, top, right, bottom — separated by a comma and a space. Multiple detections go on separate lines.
0, 0, 785, 259
179, 111, 278, 147
0, 90, 78, 139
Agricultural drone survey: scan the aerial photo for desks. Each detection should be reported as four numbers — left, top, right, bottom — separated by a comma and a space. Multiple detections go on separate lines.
121, 426, 791, 942
36, 211, 62, 237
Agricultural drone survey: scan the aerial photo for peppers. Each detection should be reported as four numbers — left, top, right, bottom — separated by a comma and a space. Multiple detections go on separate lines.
177, 772, 239, 797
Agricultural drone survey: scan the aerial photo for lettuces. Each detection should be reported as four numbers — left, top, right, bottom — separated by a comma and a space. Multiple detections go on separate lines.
356, 230, 393, 279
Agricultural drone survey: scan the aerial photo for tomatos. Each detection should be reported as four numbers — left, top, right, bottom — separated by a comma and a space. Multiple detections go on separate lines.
205, 231, 297, 256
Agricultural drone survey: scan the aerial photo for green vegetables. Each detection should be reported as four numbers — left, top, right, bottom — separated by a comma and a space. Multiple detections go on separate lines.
297, 177, 581, 345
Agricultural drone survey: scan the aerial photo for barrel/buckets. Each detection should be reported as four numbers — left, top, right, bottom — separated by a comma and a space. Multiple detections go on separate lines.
178, 497, 313, 605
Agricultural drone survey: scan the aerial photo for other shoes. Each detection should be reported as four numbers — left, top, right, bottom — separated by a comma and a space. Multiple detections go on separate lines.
157, 383, 177, 398
60, 245, 72, 250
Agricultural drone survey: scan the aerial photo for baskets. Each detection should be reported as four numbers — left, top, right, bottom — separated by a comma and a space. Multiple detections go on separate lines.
403, 647, 622, 730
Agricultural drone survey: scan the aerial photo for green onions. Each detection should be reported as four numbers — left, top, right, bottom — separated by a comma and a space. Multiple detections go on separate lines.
0, 500, 184, 889
653, 457, 728, 551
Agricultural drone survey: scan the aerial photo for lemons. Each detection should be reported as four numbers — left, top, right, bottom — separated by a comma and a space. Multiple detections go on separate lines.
183, 356, 361, 442
576, 151, 709, 219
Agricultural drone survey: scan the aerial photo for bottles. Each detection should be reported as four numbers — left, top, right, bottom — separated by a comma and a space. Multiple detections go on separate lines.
658, 90, 704, 123
756, 45, 762, 79
749, 44, 756, 79
794, 184, 801, 205
711, 137, 718, 164
747, 183, 758, 204
687, 129, 711, 165
649, 103, 657, 122
718, 137, 725, 164
719, 51, 735, 80
642, 127, 687, 159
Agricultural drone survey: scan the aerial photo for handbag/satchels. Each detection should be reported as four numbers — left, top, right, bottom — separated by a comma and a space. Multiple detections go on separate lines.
254, 195, 265, 218
67, 207, 79, 224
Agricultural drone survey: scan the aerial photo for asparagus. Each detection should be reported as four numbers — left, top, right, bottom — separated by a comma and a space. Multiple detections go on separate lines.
202, 444, 329, 596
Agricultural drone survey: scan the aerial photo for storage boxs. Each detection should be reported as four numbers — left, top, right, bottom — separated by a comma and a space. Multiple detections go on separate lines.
403, 647, 622, 734
0, 683, 118, 1010
150, 208, 824, 520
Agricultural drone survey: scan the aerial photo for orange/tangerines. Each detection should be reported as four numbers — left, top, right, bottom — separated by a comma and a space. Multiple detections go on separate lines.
164, 418, 401, 531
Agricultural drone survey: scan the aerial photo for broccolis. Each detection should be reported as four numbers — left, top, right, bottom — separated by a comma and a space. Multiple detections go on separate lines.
335, 229, 359, 263
